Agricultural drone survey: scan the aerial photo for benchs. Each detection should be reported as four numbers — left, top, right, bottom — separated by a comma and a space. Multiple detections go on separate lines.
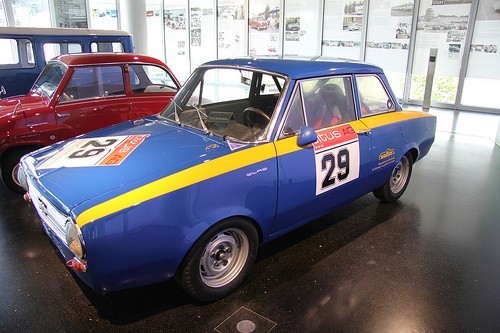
139, 84, 178, 97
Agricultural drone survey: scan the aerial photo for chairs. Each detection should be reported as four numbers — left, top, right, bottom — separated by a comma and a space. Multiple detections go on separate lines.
307, 84, 348, 135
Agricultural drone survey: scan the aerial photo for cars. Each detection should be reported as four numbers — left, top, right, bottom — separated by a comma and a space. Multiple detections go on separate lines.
64, 0, 500, 61
19, 59, 438, 302
0, 53, 184, 193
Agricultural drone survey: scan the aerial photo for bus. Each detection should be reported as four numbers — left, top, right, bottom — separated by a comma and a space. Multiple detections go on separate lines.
0, 26, 132, 99
